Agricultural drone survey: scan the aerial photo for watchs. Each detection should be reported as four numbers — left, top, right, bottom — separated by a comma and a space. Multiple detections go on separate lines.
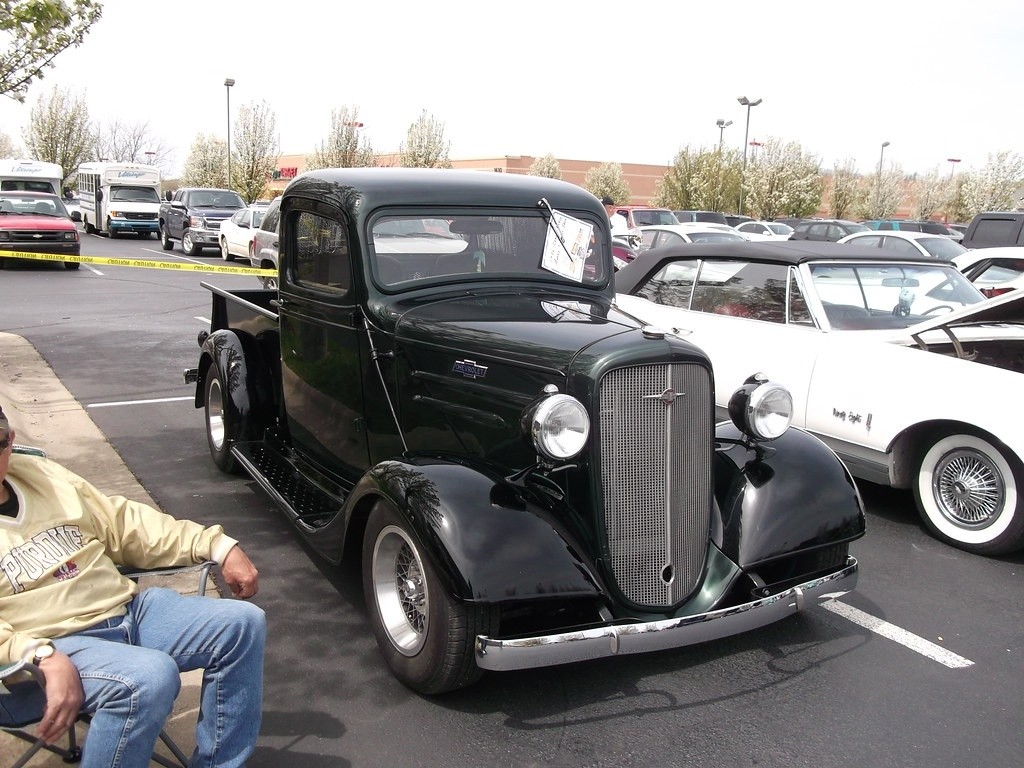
33, 644, 56, 666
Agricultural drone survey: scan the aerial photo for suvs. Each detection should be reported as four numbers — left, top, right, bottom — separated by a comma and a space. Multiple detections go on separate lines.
249, 196, 471, 292
963, 213, 1024, 248
859, 219, 949, 236
672, 211, 729, 226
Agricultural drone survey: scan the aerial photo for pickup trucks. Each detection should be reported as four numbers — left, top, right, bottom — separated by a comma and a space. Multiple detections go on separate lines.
158, 189, 247, 256
181, 164, 867, 700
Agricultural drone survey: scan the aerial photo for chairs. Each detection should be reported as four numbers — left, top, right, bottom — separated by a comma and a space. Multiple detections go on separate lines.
34, 203, 52, 213
0, 444, 218, 768
823, 305, 873, 320
751, 301, 797, 326
317, 256, 403, 289
0, 201, 17, 211
434, 252, 499, 275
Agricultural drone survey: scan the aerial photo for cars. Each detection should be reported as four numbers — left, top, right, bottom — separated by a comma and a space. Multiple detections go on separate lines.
218, 200, 269, 261
61, 197, 80, 221
605, 242, 1024, 554
946, 224, 968, 244
723, 215, 873, 243
811, 229, 1024, 299
633, 223, 751, 283
0, 190, 81, 271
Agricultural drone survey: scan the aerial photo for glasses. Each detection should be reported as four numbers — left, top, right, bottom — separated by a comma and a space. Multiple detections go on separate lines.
0, 426, 11, 451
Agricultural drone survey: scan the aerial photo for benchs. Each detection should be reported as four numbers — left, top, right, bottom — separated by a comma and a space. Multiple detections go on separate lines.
658, 285, 774, 313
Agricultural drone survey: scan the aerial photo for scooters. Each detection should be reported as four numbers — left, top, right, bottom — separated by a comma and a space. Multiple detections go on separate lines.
583, 237, 638, 283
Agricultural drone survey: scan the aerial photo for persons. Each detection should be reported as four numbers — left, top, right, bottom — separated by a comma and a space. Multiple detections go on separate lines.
0, 406, 266, 768
600, 197, 628, 239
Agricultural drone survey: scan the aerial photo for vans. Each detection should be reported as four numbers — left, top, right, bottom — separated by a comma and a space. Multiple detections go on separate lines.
612, 206, 680, 244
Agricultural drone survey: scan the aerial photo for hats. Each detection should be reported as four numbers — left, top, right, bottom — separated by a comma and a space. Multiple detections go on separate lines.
601, 196, 613, 206
0, 406, 8, 420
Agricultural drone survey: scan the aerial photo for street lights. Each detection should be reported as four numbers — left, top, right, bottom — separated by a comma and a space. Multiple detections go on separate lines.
715, 94, 961, 218
224, 78, 235, 191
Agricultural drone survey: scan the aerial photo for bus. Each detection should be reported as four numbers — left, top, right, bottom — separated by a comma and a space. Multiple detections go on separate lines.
0, 159, 69, 212
78, 163, 163, 241
